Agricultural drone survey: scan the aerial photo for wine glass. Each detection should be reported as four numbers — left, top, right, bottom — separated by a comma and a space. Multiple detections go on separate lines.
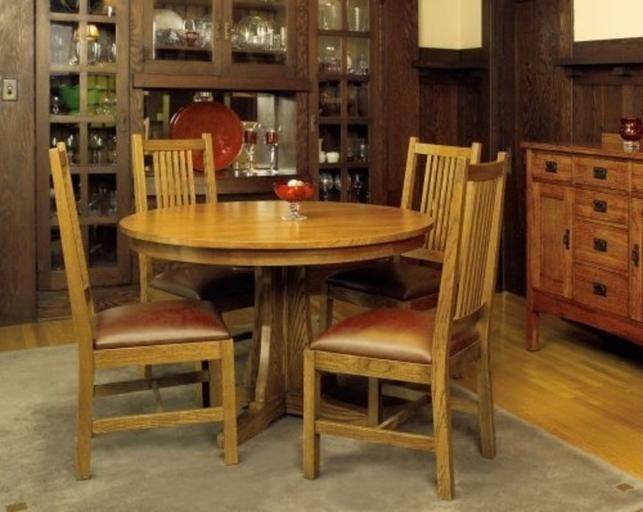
241, 121, 260, 174
272, 180, 316, 222
320, 173, 364, 201
51, 130, 117, 166
262, 125, 280, 173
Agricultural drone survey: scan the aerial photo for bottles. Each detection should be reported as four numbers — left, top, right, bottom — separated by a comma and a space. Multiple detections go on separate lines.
346, 138, 367, 163
75, 25, 100, 64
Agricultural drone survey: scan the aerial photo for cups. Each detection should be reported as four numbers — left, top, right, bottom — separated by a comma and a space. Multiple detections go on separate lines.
155, 29, 197, 47
619, 116, 643, 140
319, 151, 340, 164
321, 6, 368, 32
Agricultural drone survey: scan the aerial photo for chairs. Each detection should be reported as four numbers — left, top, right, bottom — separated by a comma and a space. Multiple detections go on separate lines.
44, 139, 243, 483
312, 130, 483, 429
295, 144, 520, 504
122, 131, 260, 412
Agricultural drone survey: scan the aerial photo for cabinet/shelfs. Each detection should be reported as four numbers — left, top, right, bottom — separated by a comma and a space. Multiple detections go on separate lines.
519, 135, 642, 363
126, 2, 311, 96
1, 1, 136, 328
123, 84, 313, 211
297, 1, 424, 299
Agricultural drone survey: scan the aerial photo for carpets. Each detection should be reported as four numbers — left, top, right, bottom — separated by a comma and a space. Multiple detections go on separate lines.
1, 314, 643, 512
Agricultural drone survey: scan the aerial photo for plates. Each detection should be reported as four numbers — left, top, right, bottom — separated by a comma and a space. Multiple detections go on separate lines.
169, 103, 243, 173
153, 7, 182, 57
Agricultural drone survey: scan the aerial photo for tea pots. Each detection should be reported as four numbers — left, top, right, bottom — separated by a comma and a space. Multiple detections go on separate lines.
49, 24, 74, 67
184, 10, 285, 52
